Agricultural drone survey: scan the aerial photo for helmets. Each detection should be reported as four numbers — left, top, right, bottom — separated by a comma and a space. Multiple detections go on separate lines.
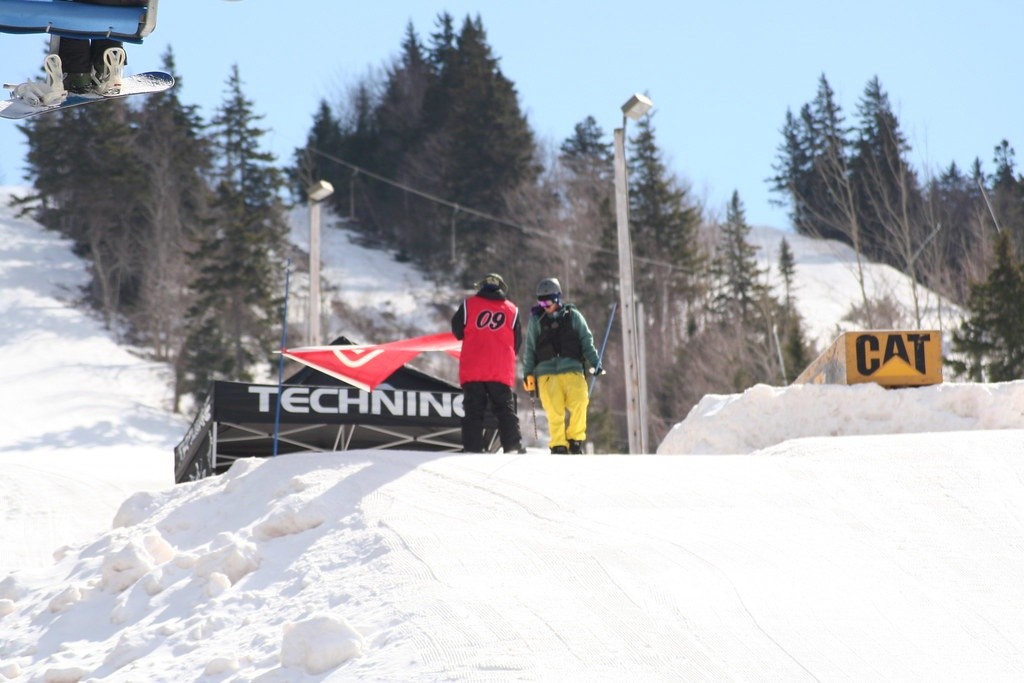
535, 278, 562, 300
473, 273, 509, 296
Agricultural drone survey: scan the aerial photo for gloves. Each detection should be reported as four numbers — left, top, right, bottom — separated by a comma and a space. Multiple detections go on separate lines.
588, 364, 607, 376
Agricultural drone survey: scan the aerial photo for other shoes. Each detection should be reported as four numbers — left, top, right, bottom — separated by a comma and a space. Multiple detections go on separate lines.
551, 446, 568, 454
504, 447, 527, 454
567, 439, 583, 454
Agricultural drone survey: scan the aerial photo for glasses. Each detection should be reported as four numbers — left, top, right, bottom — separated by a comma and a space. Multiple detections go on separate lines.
537, 300, 558, 307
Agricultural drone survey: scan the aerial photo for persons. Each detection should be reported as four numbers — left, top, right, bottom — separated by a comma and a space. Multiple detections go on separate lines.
524, 278, 606, 454
58, 35, 128, 95
451, 274, 526, 454
